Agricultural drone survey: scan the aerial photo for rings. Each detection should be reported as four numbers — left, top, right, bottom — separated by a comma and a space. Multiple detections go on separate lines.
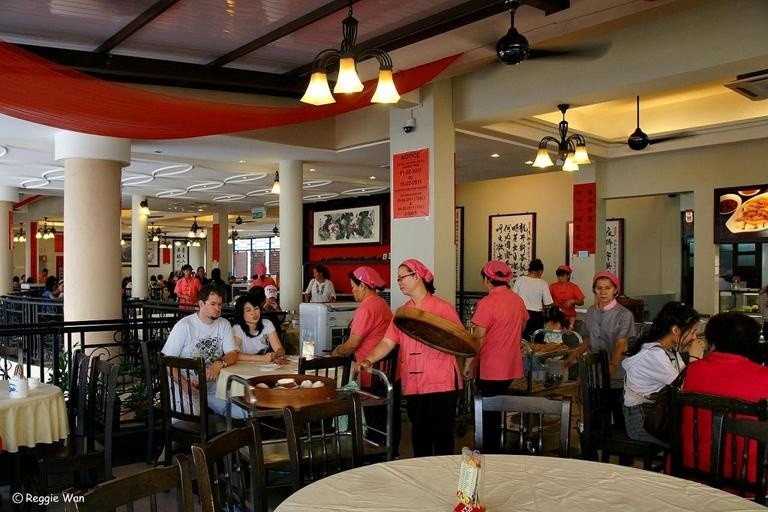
281, 356, 283, 359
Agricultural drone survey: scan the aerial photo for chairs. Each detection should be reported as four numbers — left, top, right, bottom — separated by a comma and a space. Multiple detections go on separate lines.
187, 417, 268, 512
662, 380, 766, 497
704, 407, 767, 508
471, 390, 575, 459
138, 329, 354, 490
280, 388, 366, 493
577, 346, 664, 473
55, 452, 199, 512
0, 343, 118, 510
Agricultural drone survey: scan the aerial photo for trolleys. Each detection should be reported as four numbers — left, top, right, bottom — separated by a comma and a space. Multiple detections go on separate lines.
454, 359, 476, 440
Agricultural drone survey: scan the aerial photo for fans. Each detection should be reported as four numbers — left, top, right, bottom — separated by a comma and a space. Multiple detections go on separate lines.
593, 94, 700, 151
450, 0, 610, 68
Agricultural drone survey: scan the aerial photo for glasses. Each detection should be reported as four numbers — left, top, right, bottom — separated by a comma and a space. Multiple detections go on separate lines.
397, 273, 416, 283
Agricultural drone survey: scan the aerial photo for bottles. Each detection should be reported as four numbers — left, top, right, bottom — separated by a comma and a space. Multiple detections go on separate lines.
731, 276, 740, 289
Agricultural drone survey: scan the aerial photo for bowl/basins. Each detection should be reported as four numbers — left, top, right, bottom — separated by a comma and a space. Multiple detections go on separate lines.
720, 194, 742, 214
255, 377, 324, 389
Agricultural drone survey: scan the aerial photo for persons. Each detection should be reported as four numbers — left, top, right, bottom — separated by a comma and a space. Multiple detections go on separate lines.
230, 294, 290, 366
681, 310, 768, 500
619, 300, 704, 475
330, 265, 403, 462
542, 304, 575, 345
146, 285, 255, 472
560, 271, 639, 466
549, 264, 587, 330
353, 260, 467, 457
513, 258, 554, 344
11, 261, 336, 340
463, 259, 529, 454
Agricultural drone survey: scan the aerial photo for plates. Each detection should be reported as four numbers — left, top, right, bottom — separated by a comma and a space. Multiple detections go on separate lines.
258, 364, 280, 371
725, 192, 768, 234
738, 190, 760, 196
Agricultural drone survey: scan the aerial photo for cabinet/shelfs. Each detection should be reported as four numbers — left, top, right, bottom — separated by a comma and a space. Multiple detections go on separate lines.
716, 286, 766, 347
454, 204, 465, 308
222, 368, 398, 512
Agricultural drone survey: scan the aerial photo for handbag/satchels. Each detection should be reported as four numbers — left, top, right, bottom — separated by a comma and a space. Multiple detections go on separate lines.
643, 368, 686, 441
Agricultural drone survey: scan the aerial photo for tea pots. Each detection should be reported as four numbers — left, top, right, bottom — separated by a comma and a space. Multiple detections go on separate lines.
7, 364, 28, 399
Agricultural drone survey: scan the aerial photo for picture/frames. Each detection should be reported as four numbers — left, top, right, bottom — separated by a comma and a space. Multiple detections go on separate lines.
487, 210, 537, 280
121, 235, 160, 267
563, 216, 626, 298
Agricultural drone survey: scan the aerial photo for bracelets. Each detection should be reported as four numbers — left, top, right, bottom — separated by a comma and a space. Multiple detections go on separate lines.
273, 353, 285, 358
336, 343, 343, 354
687, 354, 701, 362
216, 358, 229, 369
364, 359, 373, 366
609, 361, 618, 367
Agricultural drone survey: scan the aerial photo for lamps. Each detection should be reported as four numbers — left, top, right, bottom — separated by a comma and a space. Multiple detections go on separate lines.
293, 0, 400, 106
147, 222, 207, 250
269, 166, 281, 195
139, 198, 152, 216
35, 216, 57, 242
227, 235, 233, 245
529, 101, 592, 173
12, 218, 27, 245
188, 213, 205, 242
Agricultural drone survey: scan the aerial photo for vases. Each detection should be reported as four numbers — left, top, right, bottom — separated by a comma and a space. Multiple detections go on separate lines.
311, 204, 384, 247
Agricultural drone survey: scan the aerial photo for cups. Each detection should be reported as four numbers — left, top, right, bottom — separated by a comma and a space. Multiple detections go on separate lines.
739, 281, 747, 289
28, 377, 40, 390
